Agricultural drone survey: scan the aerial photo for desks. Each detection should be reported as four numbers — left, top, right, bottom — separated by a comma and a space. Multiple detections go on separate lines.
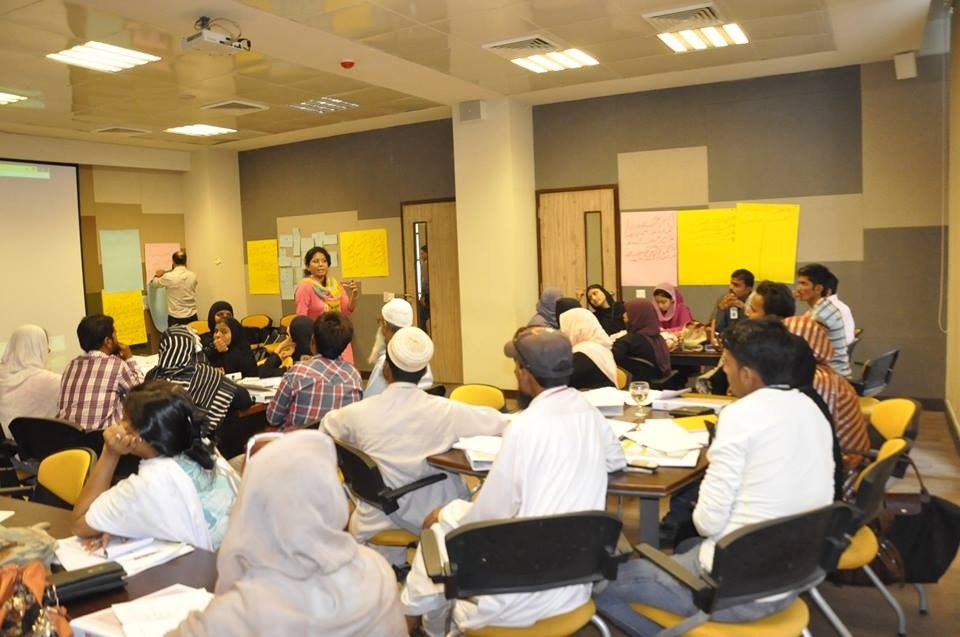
669, 346, 724, 367
0, 495, 220, 636
427, 387, 739, 558
231, 401, 268, 417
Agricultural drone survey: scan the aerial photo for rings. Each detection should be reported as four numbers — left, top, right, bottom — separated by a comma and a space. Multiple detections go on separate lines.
115, 432, 122, 440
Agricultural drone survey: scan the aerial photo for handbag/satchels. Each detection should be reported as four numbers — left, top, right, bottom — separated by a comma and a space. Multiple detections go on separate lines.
825, 453, 960, 588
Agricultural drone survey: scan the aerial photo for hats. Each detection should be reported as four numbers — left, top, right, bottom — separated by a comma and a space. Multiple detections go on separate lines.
386, 326, 435, 372
381, 298, 413, 327
503, 327, 575, 379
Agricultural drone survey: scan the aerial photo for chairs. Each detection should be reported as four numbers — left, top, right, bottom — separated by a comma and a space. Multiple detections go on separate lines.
0, 422, 43, 501
866, 395, 924, 488
324, 428, 448, 588
449, 383, 510, 414
420, 509, 624, 637
837, 436, 928, 636
634, 499, 858, 636
0, 445, 97, 509
844, 345, 903, 401
186, 313, 298, 367
8, 415, 142, 486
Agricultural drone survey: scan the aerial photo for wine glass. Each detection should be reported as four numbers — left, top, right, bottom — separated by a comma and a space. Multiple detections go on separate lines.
630, 381, 649, 416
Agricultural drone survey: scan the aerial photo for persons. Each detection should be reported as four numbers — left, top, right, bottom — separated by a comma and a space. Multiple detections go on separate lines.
71, 379, 244, 555
280, 314, 315, 368
824, 270, 855, 345
143, 322, 254, 446
207, 300, 235, 331
398, 319, 628, 637
57, 314, 144, 435
780, 317, 870, 486
592, 316, 838, 637
265, 309, 363, 433
202, 317, 258, 378
791, 263, 852, 380
280, 245, 359, 366
0, 324, 63, 442
513, 265, 797, 389
161, 427, 413, 637
315, 326, 511, 579
365, 297, 435, 395
148, 250, 199, 328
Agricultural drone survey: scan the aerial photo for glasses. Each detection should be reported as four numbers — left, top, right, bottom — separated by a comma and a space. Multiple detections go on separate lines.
376, 319, 388, 326
512, 323, 548, 368
